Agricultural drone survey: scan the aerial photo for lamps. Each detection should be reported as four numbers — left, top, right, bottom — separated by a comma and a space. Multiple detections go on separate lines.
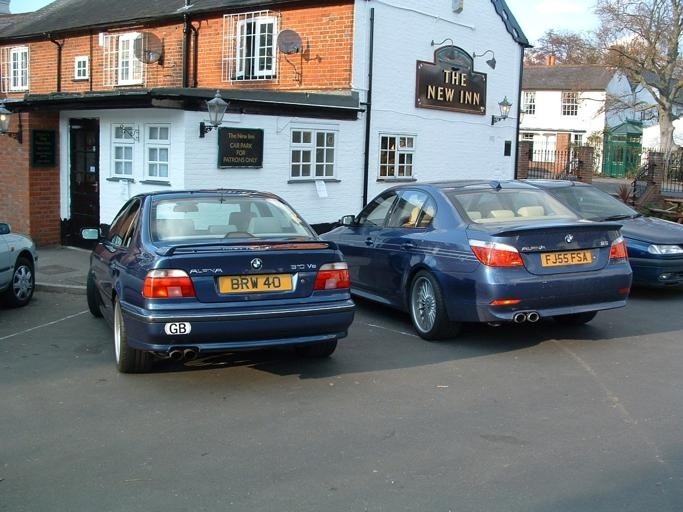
199, 88, 229, 138
472, 47, 496, 70
0, 99, 23, 144
491, 94, 512, 125
430, 38, 455, 60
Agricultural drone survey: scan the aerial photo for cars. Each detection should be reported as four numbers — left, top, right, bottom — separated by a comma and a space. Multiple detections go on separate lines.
78, 184, 358, 375
515, 175, 683, 299
317, 176, 636, 343
1, 221, 41, 309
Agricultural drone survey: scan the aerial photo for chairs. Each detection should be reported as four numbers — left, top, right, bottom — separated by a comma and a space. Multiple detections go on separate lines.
161, 212, 281, 234
409, 207, 435, 227
